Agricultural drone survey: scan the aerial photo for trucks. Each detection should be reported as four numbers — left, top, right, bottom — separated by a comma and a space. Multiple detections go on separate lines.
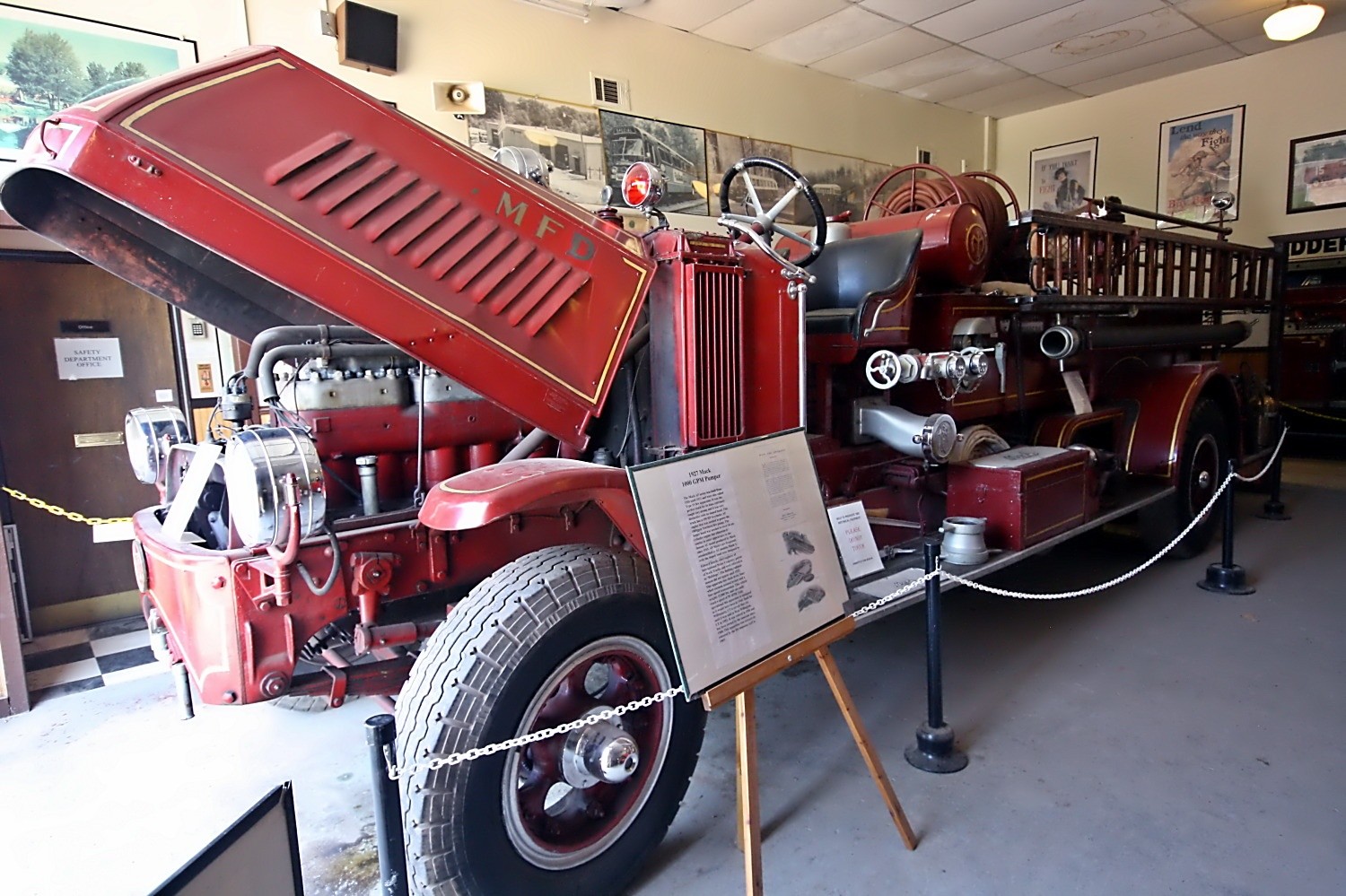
1, 39, 1299, 896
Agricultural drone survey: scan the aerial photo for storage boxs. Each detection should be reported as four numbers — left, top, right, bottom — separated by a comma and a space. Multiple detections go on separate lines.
947, 444, 1095, 550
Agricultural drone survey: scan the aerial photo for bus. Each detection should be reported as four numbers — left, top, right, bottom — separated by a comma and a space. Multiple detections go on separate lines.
605, 125, 701, 201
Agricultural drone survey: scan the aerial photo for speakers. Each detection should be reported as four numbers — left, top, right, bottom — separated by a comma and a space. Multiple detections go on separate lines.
336, 0, 399, 76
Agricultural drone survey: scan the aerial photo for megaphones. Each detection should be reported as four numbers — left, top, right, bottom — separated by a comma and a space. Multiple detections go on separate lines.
433, 80, 486, 121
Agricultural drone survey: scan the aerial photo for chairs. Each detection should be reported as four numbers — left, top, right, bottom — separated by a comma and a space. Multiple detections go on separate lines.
804, 228, 924, 347
